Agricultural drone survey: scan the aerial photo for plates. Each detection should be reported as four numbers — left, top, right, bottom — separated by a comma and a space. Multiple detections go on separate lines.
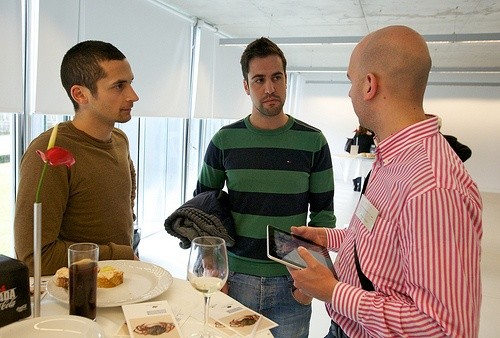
0, 314, 104, 338
46, 260, 173, 307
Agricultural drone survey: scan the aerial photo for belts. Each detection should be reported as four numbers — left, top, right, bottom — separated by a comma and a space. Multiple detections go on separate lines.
330, 319, 349, 338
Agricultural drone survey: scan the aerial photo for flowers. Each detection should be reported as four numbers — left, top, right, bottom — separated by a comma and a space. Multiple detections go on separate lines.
33, 124, 75, 204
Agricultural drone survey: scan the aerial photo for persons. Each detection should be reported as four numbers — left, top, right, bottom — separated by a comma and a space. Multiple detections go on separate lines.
14, 40, 140, 276
193, 38, 335, 338
286, 25, 482, 338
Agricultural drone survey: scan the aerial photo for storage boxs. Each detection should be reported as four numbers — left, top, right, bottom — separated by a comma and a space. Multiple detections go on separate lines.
0, 254, 30, 327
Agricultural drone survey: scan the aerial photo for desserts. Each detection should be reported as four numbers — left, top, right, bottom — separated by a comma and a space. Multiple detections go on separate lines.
98, 264, 123, 288
53, 266, 69, 288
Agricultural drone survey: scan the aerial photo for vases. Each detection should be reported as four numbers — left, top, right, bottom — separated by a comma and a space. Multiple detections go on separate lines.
34, 202, 42, 319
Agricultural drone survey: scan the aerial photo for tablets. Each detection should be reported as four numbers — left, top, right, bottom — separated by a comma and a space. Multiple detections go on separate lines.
266, 224, 340, 283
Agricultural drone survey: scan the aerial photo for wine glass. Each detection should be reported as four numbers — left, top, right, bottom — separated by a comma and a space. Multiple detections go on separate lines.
187, 236, 229, 338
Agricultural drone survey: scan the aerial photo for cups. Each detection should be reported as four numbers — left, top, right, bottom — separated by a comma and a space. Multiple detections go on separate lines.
68, 244, 99, 323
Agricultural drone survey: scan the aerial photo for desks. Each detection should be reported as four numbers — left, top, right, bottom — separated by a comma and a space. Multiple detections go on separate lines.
0, 274, 274, 338
